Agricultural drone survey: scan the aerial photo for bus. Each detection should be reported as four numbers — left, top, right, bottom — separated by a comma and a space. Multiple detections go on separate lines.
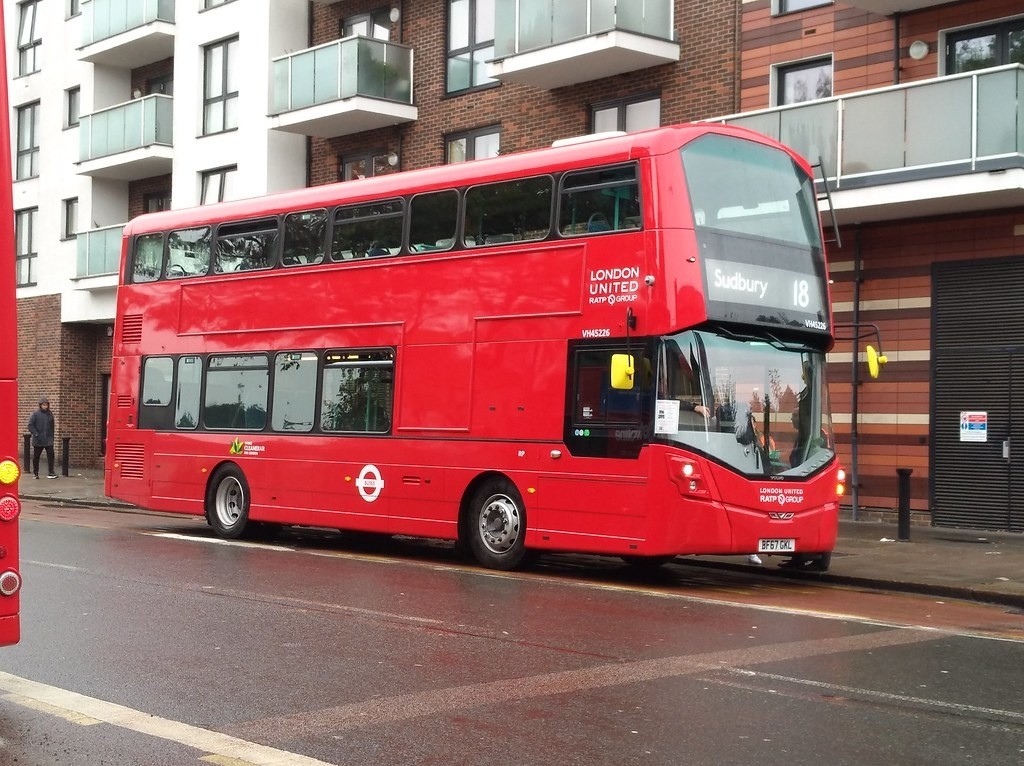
103, 122, 887, 571
0, 1, 21, 647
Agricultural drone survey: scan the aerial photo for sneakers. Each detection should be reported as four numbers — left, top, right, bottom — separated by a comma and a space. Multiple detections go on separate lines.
33, 473, 39, 480
46, 472, 58, 478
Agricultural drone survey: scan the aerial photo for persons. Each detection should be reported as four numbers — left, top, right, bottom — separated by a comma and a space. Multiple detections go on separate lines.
28, 398, 59, 479
645, 374, 710, 426
778, 407, 827, 568
744, 418, 765, 564
